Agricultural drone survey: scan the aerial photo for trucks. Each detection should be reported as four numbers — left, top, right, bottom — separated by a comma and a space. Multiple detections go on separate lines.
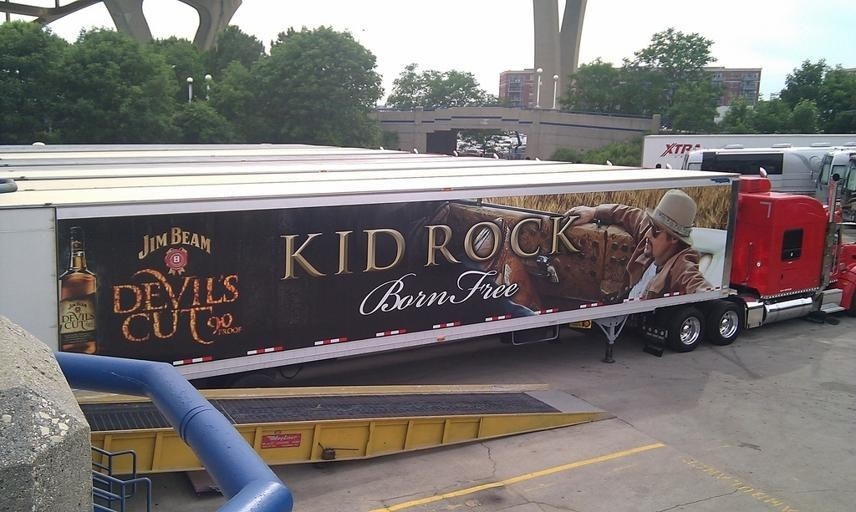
613, 167, 856, 360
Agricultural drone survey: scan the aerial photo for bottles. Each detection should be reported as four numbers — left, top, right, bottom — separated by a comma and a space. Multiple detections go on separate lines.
57, 224, 98, 358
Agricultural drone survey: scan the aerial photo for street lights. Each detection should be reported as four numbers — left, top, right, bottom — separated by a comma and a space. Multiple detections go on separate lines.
202, 73, 214, 102
536, 67, 543, 111
550, 73, 560, 109
185, 76, 194, 105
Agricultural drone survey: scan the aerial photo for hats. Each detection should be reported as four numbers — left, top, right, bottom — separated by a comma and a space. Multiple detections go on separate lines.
644, 188, 698, 247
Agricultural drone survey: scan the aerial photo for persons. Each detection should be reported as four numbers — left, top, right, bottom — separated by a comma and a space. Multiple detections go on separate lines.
563, 189, 713, 303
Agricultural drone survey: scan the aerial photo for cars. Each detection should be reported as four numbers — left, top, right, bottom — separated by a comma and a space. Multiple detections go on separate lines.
456, 133, 519, 160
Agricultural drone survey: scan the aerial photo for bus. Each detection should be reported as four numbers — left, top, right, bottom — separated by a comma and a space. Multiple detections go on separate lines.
664, 141, 856, 227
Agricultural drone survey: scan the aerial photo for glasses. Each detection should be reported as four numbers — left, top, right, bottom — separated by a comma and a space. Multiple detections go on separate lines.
647, 216, 668, 237
